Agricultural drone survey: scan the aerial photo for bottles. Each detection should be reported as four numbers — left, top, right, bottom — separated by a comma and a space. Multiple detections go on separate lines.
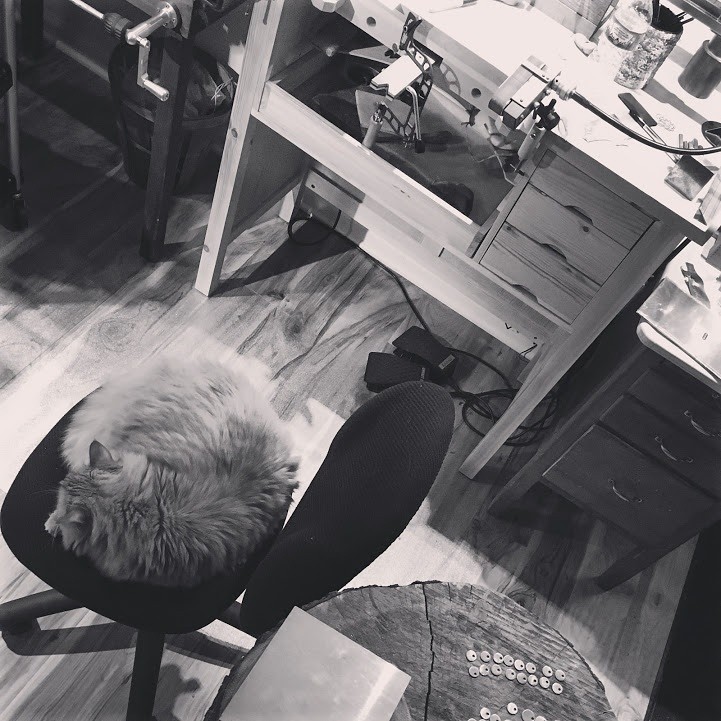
579, 0, 653, 93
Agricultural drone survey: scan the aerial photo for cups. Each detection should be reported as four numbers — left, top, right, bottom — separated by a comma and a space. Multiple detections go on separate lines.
614, 5, 683, 90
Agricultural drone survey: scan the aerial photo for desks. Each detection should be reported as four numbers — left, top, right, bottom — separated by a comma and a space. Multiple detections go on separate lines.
212, 579, 615, 721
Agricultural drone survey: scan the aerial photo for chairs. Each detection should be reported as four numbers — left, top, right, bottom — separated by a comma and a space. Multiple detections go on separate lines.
0, 383, 456, 720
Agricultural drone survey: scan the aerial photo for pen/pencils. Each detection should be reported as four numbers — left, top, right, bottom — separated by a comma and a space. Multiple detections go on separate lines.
673, 10, 695, 25
628, 108, 678, 163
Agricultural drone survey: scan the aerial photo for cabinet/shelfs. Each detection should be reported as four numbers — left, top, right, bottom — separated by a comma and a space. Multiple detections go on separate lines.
486, 319, 721, 588
192, 1, 709, 476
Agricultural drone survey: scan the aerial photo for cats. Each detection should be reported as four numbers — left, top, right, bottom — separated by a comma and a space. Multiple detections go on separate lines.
43, 356, 301, 590
